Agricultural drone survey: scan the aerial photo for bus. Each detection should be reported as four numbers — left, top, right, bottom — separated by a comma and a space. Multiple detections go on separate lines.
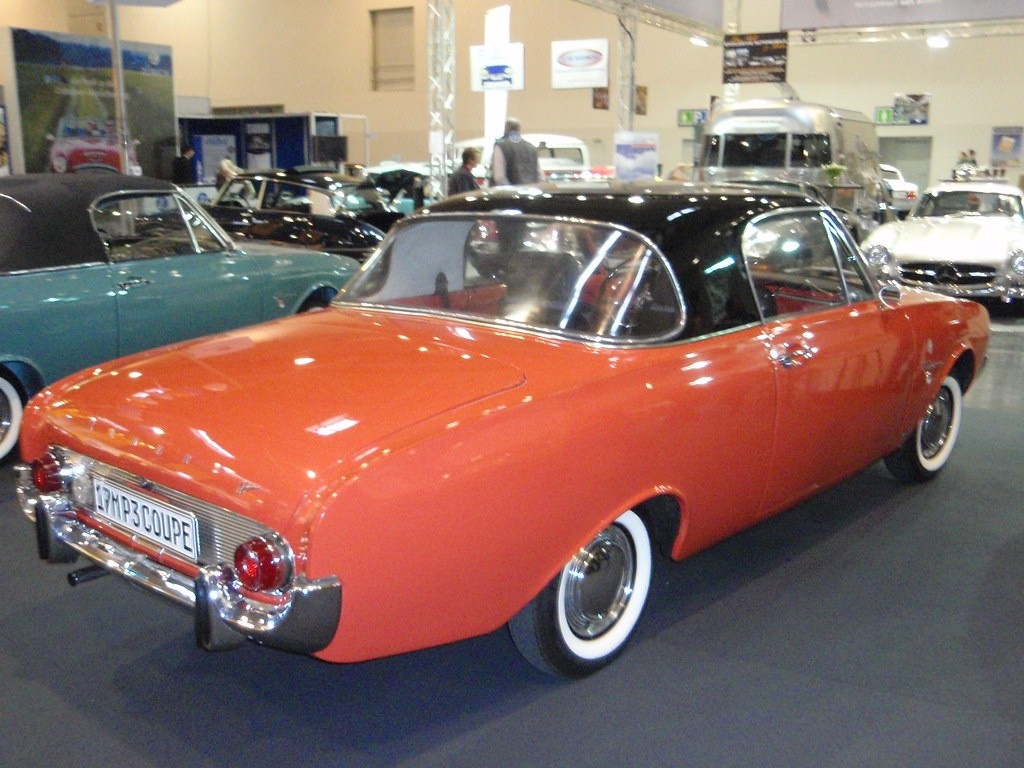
701, 102, 879, 232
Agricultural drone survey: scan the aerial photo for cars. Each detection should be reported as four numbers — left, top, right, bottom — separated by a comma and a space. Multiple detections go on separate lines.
50, 116, 135, 175
878, 164, 918, 219
365, 165, 459, 214
16, 177, 990, 680
860, 180, 1024, 312
195, 169, 407, 265
0, 172, 364, 467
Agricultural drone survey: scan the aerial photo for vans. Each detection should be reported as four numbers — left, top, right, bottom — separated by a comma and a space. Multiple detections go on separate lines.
457, 134, 588, 186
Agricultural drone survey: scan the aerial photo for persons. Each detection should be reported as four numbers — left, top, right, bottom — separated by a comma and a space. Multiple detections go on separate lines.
215, 159, 254, 194
172, 144, 197, 185
448, 147, 482, 196
489, 118, 538, 188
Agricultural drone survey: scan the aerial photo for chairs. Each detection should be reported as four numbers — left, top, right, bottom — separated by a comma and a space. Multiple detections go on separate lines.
497, 248, 579, 327
